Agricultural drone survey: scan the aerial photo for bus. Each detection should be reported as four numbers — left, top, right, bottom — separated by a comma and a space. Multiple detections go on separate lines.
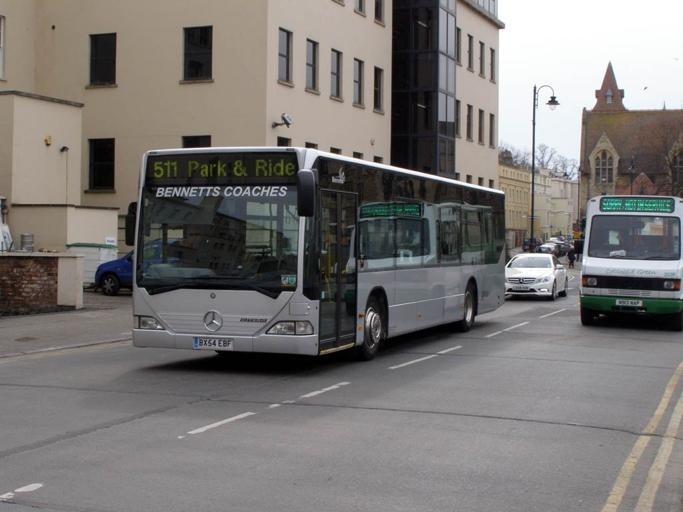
342, 198, 497, 317
132, 141, 506, 364
579, 193, 682, 331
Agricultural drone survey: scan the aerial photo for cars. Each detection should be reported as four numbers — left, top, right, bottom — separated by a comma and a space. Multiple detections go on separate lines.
93, 237, 185, 297
523, 234, 573, 258
502, 253, 569, 302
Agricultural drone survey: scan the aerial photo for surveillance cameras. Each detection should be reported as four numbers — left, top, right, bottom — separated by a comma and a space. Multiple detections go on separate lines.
272, 113, 292, 129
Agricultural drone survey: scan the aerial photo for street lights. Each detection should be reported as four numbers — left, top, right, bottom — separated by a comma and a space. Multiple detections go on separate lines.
529, 83, 559, 253
628, 156, 638, 195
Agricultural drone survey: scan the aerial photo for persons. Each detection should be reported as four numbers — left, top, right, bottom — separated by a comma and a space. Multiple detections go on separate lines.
567, 246, 576, 269
